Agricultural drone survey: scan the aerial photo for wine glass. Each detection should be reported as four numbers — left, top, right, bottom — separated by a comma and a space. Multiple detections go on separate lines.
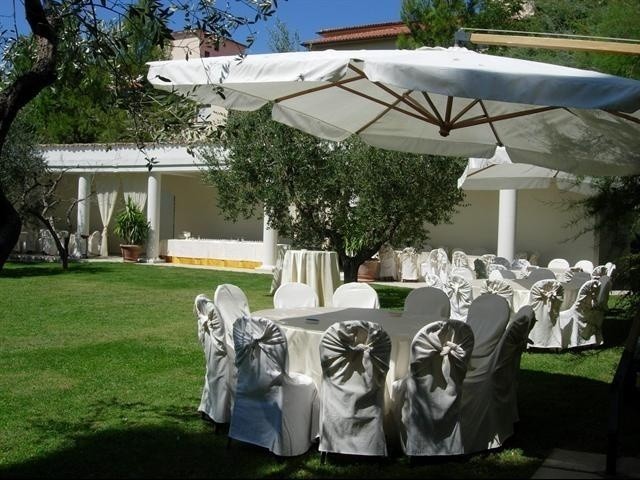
184, 232, 191, 240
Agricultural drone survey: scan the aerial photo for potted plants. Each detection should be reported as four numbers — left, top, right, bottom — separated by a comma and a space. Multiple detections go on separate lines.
112, 195, 152, 263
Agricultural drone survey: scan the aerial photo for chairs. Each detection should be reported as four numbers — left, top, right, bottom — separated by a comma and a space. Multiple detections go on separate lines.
12, 228, 100, 260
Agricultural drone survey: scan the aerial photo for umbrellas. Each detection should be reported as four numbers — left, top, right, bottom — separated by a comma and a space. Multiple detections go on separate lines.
457, 146, 639, 209
144, 28, 640, 178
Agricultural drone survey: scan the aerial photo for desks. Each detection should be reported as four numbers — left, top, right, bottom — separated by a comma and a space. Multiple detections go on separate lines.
158, 238, 291, 272
